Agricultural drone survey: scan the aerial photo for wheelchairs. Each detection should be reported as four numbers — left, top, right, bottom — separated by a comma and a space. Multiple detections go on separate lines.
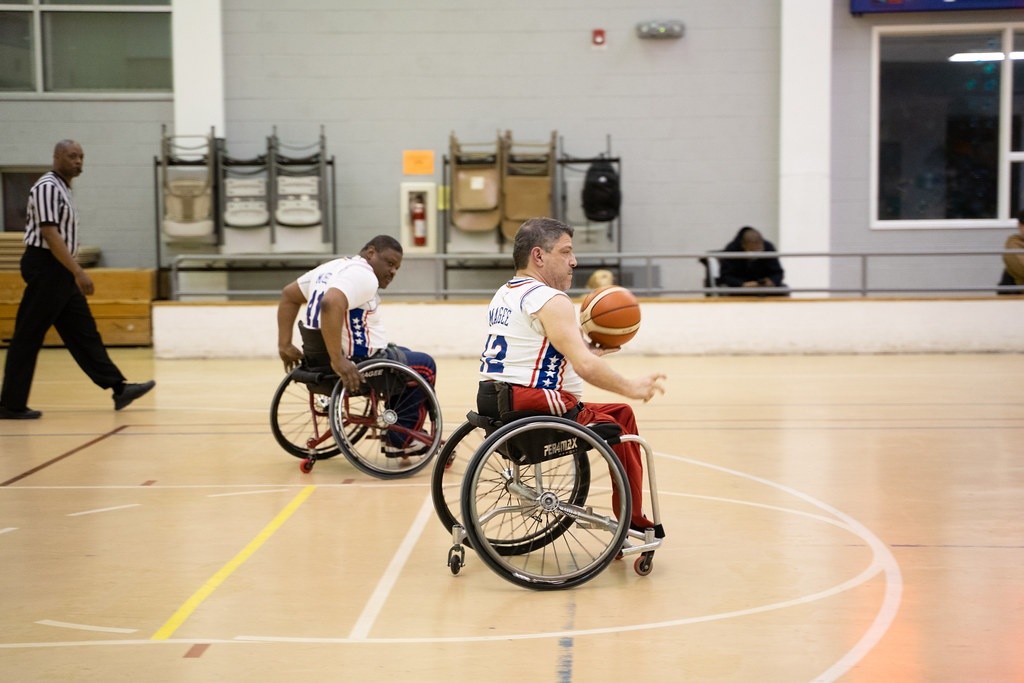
270, 320, 457, 481
427, 379, 666, 593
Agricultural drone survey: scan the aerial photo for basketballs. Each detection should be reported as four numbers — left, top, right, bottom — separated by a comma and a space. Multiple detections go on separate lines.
579, 284, 642, 348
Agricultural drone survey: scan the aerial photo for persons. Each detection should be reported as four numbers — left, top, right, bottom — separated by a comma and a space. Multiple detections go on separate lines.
276, 235, 437, 459
994, 210, 1024, 299
719, 227, 785, 296
0, 140, 156, 420
478, 216, 667, 532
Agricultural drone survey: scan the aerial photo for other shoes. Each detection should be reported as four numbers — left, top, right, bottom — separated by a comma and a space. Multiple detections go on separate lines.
112, 380, 156, 410
0, 406, 42, 419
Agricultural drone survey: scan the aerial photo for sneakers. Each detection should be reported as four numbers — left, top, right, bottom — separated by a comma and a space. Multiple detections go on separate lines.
385, 440, 428, 458
380, 440, 385, 453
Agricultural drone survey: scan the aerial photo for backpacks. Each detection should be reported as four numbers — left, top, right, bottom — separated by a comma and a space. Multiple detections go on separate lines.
581, 161, 622, 240
